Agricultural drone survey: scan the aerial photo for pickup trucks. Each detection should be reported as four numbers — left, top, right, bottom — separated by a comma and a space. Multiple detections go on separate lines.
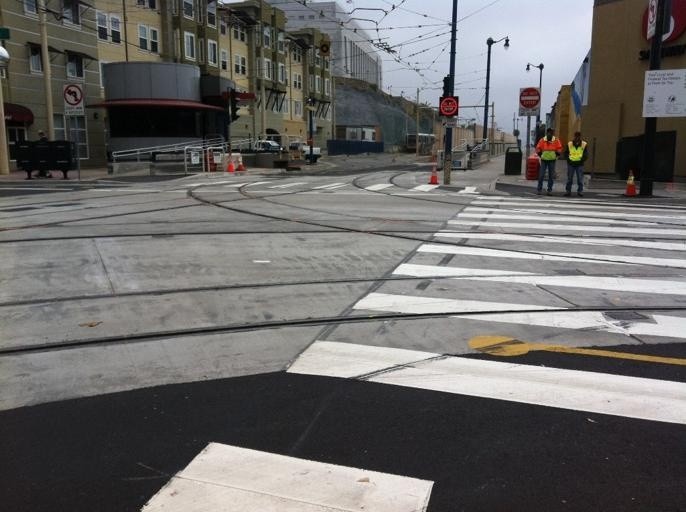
286, 141, 321, 163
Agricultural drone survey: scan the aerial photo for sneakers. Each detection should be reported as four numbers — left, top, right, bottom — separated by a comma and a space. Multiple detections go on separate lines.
537, 191, 552, 196
563, 192, 571, 196
578, 192, 584, 196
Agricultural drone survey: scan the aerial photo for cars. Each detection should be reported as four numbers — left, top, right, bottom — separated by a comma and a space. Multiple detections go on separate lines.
253, 140, 284, 151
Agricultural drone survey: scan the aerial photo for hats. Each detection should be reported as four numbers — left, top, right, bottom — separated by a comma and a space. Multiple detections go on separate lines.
547, 128, 555, 132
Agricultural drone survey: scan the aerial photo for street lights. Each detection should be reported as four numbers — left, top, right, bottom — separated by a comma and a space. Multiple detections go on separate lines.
307, 95, 313, 162
526, 63, 544, 158
482, 35, 510, 149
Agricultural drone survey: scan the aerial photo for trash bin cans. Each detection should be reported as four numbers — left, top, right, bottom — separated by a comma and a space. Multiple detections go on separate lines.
504, 147, 523, 175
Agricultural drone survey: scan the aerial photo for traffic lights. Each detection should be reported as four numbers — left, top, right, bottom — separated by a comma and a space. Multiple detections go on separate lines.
228, 86, 240, 124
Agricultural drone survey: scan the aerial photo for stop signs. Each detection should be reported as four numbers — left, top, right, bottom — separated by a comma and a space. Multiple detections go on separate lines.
519, 86, 540, 109
439, 95, 459, 117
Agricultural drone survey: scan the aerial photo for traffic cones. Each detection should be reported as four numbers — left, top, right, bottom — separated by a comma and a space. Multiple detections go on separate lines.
225, 155, 236, 172
236, 157, 246, 172
622, 170, 640, 197
428, 166, 440, 184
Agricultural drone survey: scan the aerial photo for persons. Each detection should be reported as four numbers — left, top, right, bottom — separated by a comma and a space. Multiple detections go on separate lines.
535, 127, 563, 196
563, 131, 590, 197
36, 129, 47, 141
